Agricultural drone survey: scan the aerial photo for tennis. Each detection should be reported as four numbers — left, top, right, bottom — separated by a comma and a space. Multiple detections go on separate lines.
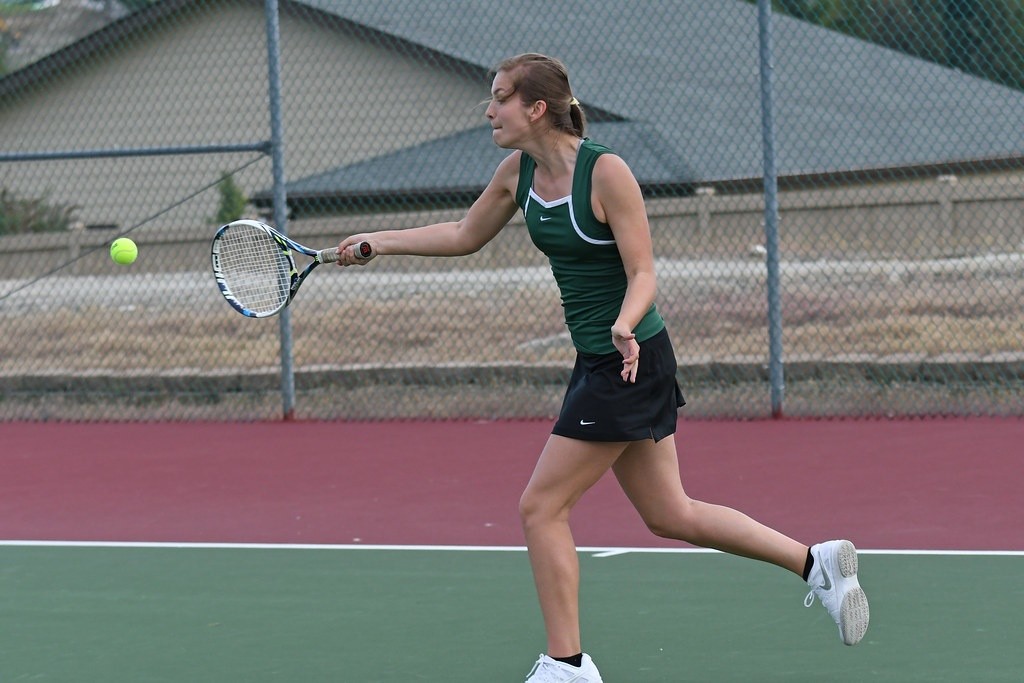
109, 237, 138, 266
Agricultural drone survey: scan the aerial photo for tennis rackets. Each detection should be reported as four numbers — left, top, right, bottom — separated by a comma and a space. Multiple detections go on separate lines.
211, 217, 373, 319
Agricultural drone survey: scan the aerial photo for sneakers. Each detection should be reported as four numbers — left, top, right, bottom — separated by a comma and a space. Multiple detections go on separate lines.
803, 539, 870, 646
524, 652, 603, 683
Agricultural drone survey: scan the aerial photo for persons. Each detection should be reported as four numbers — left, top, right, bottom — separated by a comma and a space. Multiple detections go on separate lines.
336, 52, 870, 683
